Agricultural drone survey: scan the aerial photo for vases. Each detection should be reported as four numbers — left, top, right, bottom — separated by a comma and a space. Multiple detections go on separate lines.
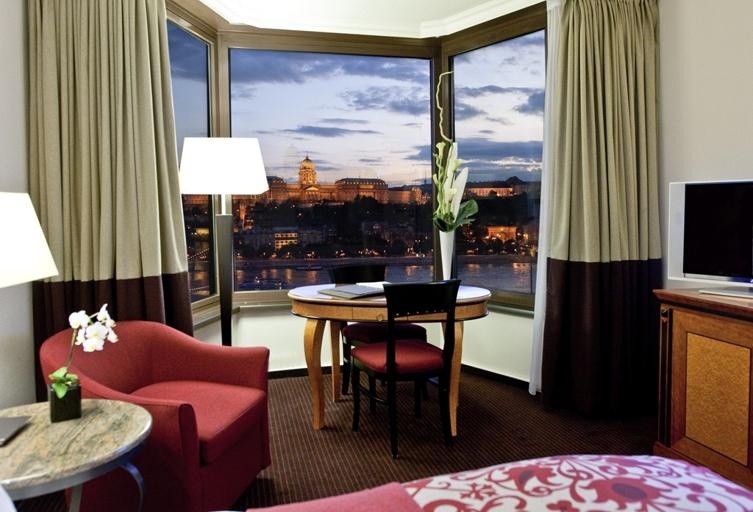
436, 228, 456, 284
43, 381, 86, 423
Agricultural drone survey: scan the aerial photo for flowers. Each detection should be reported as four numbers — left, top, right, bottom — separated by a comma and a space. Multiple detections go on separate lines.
422, 67, 481, 234
45, 299, 120, 403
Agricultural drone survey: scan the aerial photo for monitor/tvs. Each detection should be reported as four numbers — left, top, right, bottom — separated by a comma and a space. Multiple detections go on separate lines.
667, 180, 753, 299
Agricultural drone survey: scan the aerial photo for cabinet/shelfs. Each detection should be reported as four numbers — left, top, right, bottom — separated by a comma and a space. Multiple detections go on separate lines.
649, 283, 752, 494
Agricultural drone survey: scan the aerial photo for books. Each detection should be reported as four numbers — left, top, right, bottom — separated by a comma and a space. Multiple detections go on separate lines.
319, 283, 385, 300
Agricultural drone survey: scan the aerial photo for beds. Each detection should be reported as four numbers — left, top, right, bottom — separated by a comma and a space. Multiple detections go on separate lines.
194, 449, 749, 512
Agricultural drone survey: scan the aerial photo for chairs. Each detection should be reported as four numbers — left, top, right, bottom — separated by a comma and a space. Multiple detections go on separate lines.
346, 276, 462, 460
323, 259, 429, 414
36, 315, 279, 511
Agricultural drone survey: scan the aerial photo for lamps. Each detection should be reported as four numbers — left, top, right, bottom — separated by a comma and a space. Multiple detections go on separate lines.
0, 188, 61, 294
171, 135, 272, 346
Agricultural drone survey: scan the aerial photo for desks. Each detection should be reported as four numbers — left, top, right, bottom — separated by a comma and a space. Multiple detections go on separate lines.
0, 393, 155, 510
284, 277, 494, 440
